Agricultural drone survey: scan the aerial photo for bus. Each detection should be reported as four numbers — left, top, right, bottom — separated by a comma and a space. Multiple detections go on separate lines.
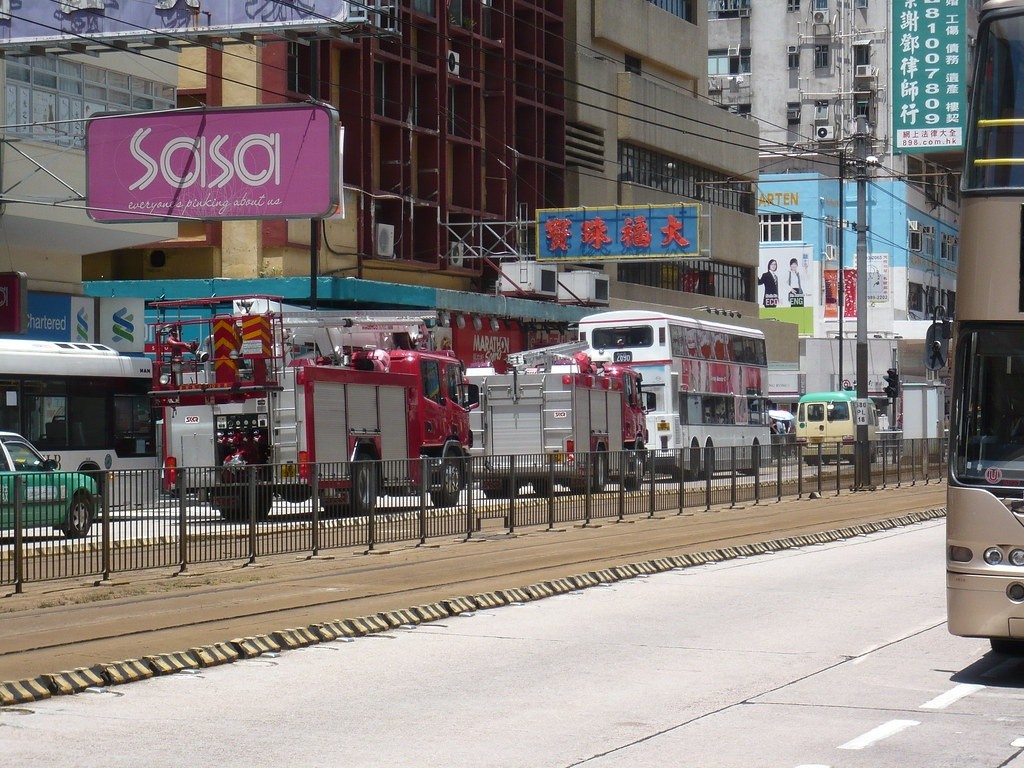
0, 337, 161, 506
577, 310, 773, 479
921, 0, 1024, 650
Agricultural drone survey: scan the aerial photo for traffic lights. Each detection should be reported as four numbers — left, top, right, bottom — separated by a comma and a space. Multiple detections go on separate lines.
882, 369, 899, 397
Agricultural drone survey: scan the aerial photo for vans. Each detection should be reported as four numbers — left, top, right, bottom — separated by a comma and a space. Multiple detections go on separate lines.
795, 391, 881, 466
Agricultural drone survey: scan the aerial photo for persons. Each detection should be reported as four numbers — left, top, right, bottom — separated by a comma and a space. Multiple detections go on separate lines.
786, 259, 805, 308
757, 260, 779, 308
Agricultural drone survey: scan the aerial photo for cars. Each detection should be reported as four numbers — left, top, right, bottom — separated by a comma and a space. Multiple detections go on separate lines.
0, 430, 102, 539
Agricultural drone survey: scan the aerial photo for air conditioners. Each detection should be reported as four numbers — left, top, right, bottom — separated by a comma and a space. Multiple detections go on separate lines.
817, 126, 833, 139
448, 241, 464, 267
856, 65, 872, 77
448, 49, 460, 76
812, 10, 830, 25
376, 223, 394, 258
909, 231, 922, 252
826, 244, 836, 262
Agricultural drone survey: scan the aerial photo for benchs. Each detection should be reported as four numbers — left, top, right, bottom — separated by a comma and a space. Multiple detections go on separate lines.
45, 422, 86, 446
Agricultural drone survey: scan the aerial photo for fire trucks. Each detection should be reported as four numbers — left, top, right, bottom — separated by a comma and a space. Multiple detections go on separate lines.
460, 339, 657, 499
146, 294, 481, 520
